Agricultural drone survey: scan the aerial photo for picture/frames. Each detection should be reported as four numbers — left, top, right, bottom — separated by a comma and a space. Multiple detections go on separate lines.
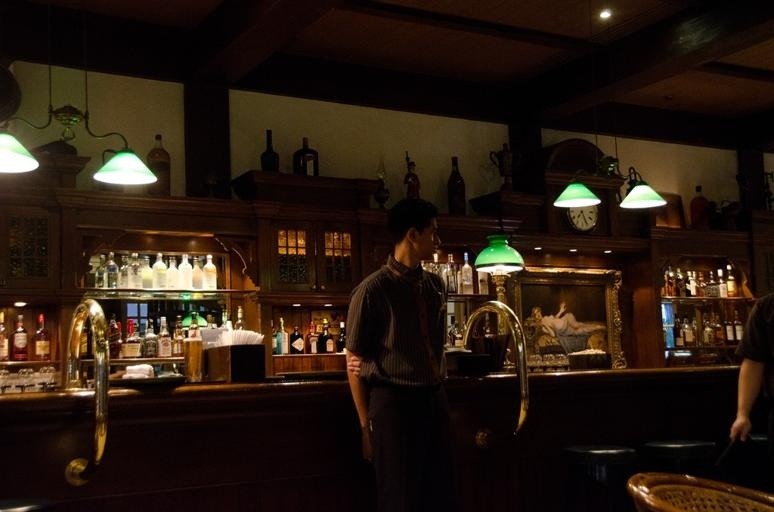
656, 191, 684, 229
510, 264, 628, 373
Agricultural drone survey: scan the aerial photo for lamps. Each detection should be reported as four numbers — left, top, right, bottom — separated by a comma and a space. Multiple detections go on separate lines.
0, 64, 53, 174
552, 163, 602, 208
85, 71, 159, 186
614, 137, 669, 210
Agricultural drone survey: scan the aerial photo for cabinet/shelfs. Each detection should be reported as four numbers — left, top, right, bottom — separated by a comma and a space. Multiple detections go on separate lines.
656, 255, 753, 350
245, 298, 350, 379
362, 207, 522, 296
260, 199, 360, 297
1, 185, 61, 290
62, 188, 259, 292
0, 292, 232, 391
445, 299, 497, 349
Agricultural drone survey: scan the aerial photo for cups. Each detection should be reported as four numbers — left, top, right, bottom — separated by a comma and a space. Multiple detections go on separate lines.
182, 338, 204, 384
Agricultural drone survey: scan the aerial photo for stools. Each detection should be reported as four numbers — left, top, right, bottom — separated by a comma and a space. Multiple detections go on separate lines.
562, 445, 636, 511
643, 440, 715, 472
748, 435, 774, 470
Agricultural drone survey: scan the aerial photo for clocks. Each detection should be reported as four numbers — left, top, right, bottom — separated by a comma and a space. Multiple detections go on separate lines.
567, 205, 599, 232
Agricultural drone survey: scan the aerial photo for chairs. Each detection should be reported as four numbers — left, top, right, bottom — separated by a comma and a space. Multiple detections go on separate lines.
626, 470, 773, 511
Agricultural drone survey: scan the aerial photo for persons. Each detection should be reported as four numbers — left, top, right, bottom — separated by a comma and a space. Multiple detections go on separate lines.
345, 197, 448, 511
729, 292, 774, 443
531, 302, 606, 338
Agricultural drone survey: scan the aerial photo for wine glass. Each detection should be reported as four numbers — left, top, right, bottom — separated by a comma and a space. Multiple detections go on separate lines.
530, 353, 566, 373
0, 366, 56, 393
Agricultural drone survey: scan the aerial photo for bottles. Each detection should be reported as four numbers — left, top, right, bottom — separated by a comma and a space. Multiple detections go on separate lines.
260, 129, 279, 172
447, 156, 465, 218
664, 261, 745, 348
271, 250, 474, 356
292, 137, 320, 176
146, 134, 171, 197
0, 249, 246, 361
690, 186, 711, 230
